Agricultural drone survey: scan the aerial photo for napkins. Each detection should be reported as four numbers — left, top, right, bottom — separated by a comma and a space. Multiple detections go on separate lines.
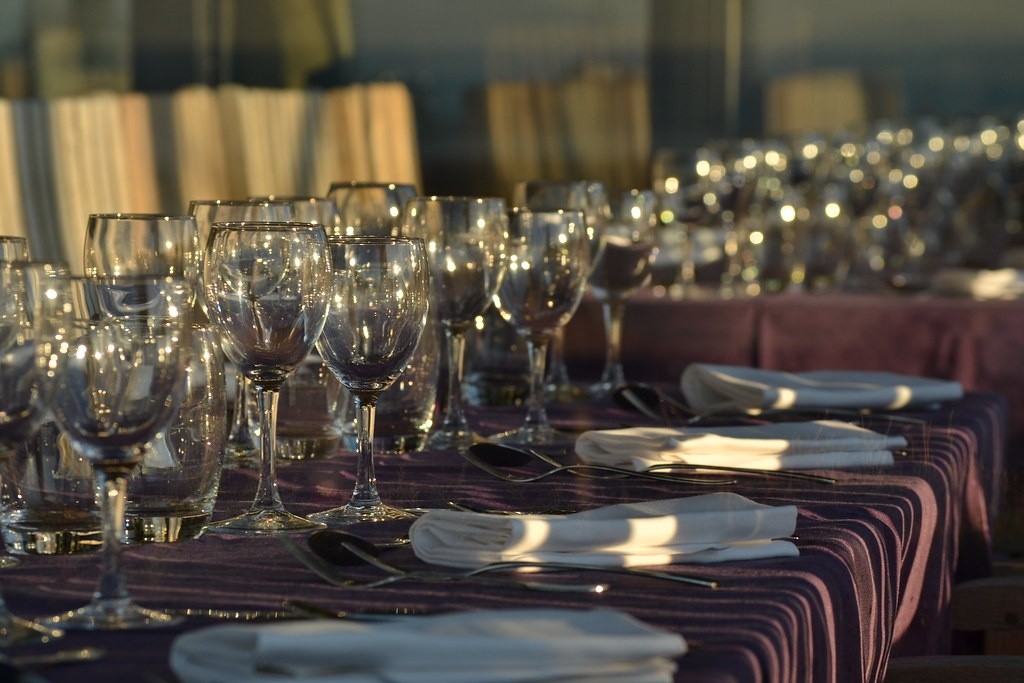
576, 417, 905, 481
169, 606, 686, 683
676, 362, 965, 418
407, 493, 803, 568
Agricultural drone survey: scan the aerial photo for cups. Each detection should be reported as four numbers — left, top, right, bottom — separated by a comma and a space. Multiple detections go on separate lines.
249, 181, 425, 238
249, 319, 439, 462
86, 324, 226, 541
0, 413, 108, 554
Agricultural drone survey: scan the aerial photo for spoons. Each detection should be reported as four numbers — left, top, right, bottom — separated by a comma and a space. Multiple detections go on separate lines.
614, 383, 666, 407
468, 440, 575, 467
307, 527, 414, 563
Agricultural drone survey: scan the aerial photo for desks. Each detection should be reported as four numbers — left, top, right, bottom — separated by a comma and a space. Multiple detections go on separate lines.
562, 288, 1024, 482
0, 370, 1007, 683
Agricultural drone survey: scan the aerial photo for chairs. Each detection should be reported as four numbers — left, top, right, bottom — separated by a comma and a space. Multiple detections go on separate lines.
0, 92, 156, 269
166, 80, 419, 219
757, 69, 865, 143
464, 79, 651, 201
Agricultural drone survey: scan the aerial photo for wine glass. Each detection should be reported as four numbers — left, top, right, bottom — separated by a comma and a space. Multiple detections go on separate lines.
584, 188, 660, 393
307, 235, 428, 530
519, 179, 608, 414
207, 220, 325, 538
189, 195, 296, 468
404, 194, 508, 452
0, 211, 201, 667
483, 209, 581, 452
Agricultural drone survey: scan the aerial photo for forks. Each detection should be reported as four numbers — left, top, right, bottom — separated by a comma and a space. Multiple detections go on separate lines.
529, 447, 837, 484
653, 388, 924, 430
279, 537, 610, 592
460, 450, 737, 486
340, 541, 718, 588
